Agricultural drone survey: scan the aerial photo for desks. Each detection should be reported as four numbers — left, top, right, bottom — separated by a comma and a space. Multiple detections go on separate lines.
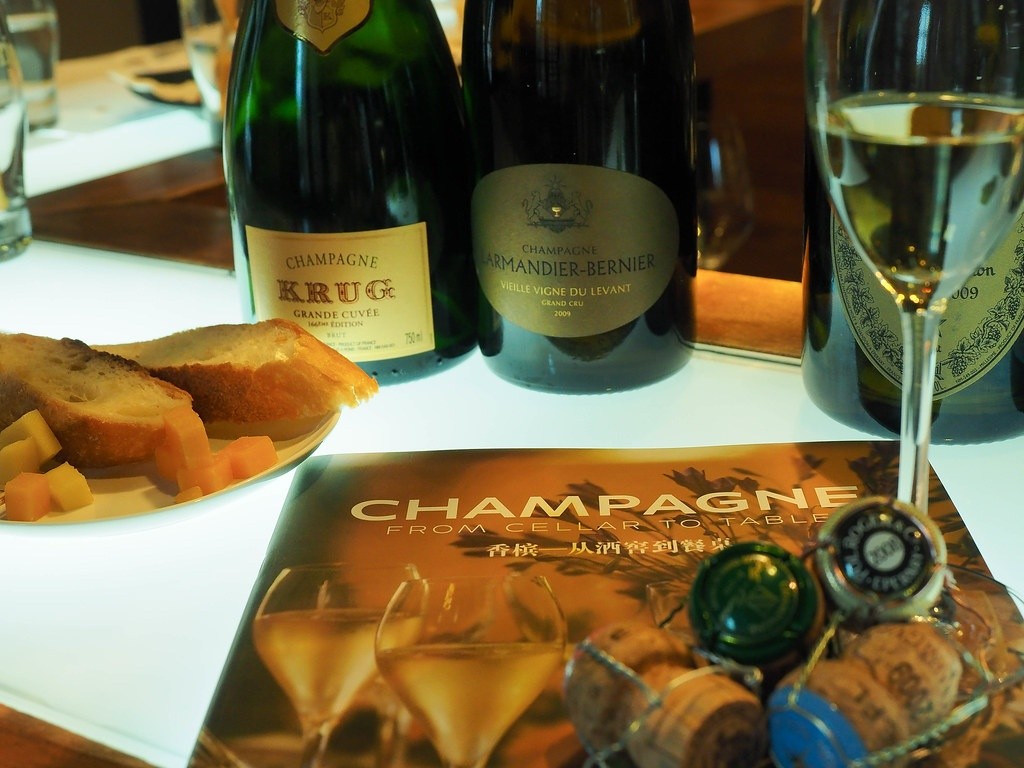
0, 2, 805, 768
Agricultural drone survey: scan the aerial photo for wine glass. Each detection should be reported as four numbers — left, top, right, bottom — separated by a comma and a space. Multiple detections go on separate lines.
252, 566, 417, 768
810, 0, 1024, 671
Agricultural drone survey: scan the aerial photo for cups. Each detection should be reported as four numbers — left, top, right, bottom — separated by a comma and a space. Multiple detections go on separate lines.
0, 0, 57, 132
375, 576, 565, 768
178, 0, 242, 117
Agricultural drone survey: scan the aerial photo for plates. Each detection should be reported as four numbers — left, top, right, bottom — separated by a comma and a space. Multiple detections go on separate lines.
0, 404, 339, 538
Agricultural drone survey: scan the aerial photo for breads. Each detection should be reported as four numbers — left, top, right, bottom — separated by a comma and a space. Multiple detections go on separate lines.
1, 332, 193, 459
88, 319, 375, 422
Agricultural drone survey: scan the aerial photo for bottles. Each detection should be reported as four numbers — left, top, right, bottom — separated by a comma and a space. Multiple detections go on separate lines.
804, 0, 1024, 447
463, 0, 703, 394
221, 0, 478, 386
0, 0, 32, 261
